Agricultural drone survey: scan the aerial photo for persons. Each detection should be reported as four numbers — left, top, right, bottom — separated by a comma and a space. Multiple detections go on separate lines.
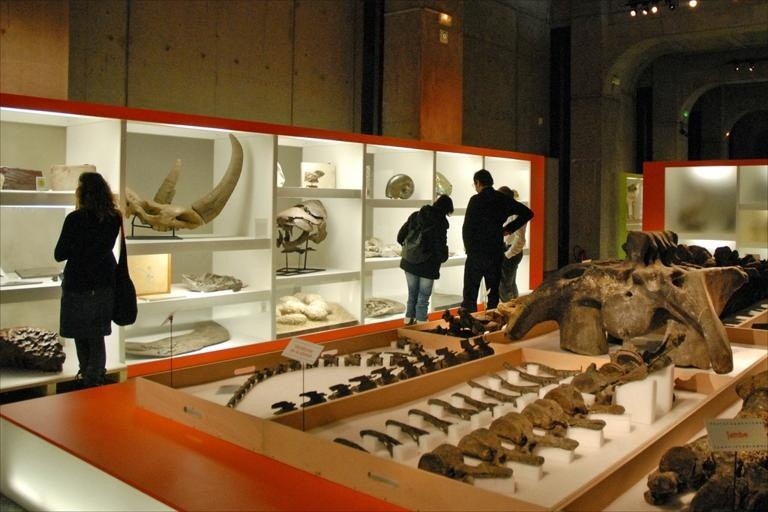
497, 185, 529, 303
53, 171, 122, 389
396, 194, 453, 328
458, 168, 534, 314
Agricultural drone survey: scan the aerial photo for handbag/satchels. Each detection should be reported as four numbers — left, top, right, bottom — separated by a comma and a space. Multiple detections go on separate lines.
112, 264, 137, 326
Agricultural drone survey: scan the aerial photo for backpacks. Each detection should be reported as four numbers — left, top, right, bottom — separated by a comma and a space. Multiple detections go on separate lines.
399, 211, 442, 265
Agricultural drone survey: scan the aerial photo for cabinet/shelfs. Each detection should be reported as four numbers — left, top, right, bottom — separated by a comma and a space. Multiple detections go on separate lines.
644, 158, 768, 262
616, 172, 643, 259
1, 92, 126, 398
123, 106, 544, 387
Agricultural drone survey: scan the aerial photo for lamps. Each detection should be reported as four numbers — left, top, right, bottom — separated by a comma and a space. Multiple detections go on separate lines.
630, 1, 678, 17
